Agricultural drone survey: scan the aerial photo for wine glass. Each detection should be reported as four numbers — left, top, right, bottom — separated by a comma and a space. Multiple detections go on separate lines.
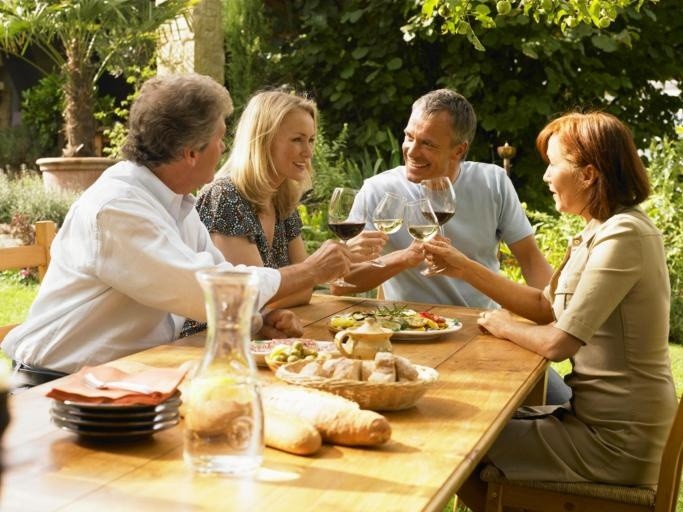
404, 198, 449, 278
326, 187, 369, 288
366, 190, 408, 269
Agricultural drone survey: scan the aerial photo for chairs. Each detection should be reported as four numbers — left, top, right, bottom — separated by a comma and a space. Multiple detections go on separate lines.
0, 222, 62, 346
479, 387, 683, 511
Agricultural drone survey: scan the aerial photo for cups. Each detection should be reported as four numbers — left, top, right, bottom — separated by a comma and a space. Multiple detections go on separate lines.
417, 175, 457, 238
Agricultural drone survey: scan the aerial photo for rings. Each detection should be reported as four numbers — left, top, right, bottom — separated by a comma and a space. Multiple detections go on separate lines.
482, 311, 489, 319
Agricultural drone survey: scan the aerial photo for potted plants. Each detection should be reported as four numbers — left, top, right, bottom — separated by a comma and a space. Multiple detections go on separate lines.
0, 0, 198, 195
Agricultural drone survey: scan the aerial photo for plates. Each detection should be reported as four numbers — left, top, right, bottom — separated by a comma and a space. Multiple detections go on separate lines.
47, 388, 183, 444
328, 311, 463, 341
251, 338, 321, 369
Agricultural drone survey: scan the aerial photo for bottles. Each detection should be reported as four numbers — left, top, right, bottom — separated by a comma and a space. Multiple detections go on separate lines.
183, 266, 267, 478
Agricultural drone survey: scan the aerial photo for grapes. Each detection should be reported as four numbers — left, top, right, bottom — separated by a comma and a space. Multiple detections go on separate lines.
274, 341, 318, 362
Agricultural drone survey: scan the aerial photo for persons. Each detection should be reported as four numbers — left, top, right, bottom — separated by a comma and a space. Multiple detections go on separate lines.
329, 89, 573, 406
178, 89, 389, 342
0, 70, 351, 398
417, 109, 677, 511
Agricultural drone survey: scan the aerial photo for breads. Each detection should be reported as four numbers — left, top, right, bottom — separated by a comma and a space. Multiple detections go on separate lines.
185, 382, 392, 456
302, 351, 419, 383
